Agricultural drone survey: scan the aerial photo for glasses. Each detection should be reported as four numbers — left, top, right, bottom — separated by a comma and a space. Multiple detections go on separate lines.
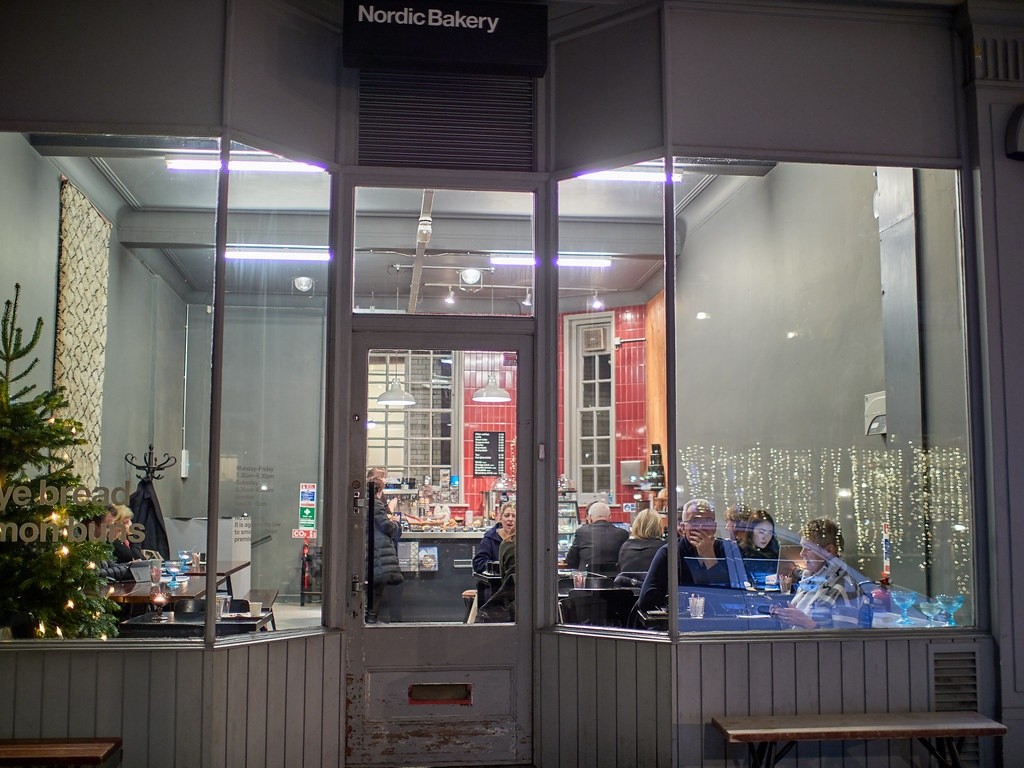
683, 518, 715, 527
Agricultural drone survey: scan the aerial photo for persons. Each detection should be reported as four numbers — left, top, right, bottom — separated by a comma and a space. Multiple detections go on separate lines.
367, 479, 402, 623
474, 522, 515, 623
77, 500, 146, 578
565, 500, 779, 611
769, 518, 863, 630
472, 501, 516, 606
369, 467, 392, 515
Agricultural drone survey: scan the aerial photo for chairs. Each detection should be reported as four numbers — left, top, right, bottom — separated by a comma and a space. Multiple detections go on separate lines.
462, 573, 664, 630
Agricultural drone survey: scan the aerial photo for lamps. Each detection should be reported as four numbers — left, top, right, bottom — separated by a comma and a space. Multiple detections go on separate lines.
443, 286, 455, 305
377, 353, 416, 406
292, 275, 315, 298
521, 290, 534, 307
471, 352, 512, 403
592, 290, 602, 309
457, 269, 485, 293
580, 165, 684, 183
224, 249, 331, 261
164, 154, 329, 173
490, 253, 614, 268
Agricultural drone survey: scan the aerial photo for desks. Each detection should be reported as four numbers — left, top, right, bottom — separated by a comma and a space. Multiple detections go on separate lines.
118, 611, 274, 633
106, 582, 147, 602
162, 555, 251, 598
635, 607, 669, 632
471, 572, 622, 608
124, 574, 227, 616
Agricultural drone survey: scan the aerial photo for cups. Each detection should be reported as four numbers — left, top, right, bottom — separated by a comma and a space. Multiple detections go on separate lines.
192, 552, 200, 567
486, 563, 499, 574
150, 564, 161, 586
249, 602, 262, 617
779, 578, 792, 595
216, 595, 232, 622
571, 570, 587, 589
688, 597, 705, 619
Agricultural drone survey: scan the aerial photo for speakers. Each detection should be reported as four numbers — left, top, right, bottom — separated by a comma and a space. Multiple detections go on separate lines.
620, 460, 644, 485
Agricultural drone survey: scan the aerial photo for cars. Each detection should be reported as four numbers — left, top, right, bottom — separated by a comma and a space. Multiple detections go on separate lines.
673, 520, 950, 628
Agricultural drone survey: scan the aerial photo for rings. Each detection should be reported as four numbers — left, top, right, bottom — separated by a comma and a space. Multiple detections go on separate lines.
785, 616, 787, 620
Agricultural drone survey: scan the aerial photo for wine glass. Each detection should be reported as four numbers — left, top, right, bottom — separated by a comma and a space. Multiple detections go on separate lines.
165, 560, 187, 587
891, 591, 917, 625
177, 550, 193, 570
919, 594, 964, 627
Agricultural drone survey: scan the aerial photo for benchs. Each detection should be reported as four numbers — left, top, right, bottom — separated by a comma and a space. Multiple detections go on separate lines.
0, 736, 122, 768
714, 709, 1008, 768
244, 585, 280, 632
